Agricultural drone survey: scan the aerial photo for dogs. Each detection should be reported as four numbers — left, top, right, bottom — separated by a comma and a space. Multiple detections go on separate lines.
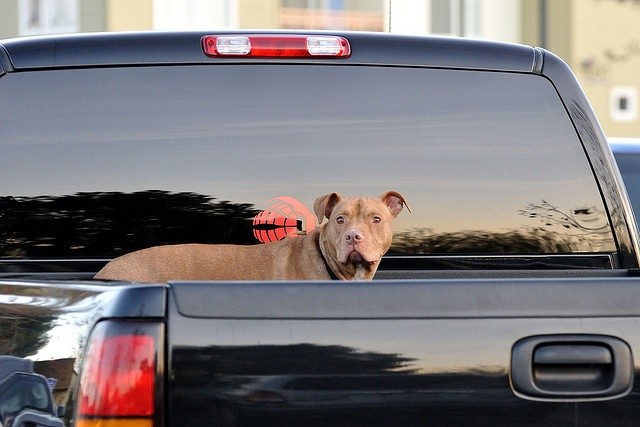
91, 189, 413, 283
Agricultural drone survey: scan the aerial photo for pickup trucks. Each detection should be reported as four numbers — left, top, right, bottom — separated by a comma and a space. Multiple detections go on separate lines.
0, 31, 640, 422
